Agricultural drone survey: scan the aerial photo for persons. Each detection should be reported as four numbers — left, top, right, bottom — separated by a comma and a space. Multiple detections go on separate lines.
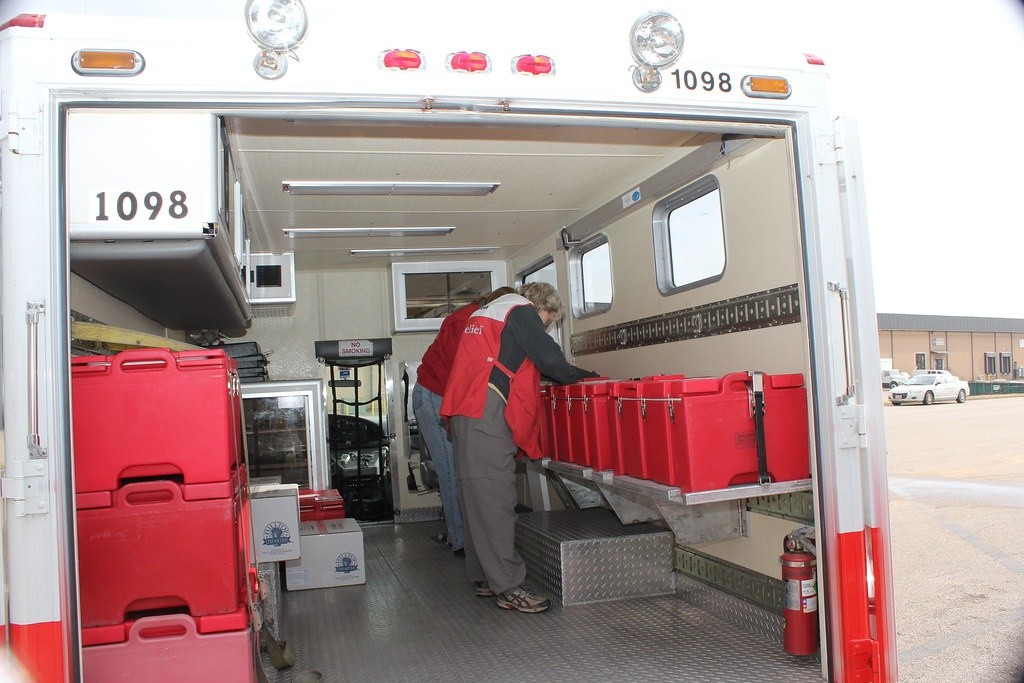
439, 282, 601, 614
412, 286, 518, 558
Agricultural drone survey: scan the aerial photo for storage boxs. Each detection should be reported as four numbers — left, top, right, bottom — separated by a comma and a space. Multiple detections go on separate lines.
539, 369, 805, 492
72, 350, 365, 682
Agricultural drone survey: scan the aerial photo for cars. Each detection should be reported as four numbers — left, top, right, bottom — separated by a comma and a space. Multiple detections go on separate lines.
886, 374, 970, 405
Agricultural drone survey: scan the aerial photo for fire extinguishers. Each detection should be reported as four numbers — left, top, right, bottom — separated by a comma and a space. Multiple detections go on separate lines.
779, 526, 817, 657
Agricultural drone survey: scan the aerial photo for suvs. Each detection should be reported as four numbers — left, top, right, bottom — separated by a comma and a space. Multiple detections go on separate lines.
882, 368, 905, 389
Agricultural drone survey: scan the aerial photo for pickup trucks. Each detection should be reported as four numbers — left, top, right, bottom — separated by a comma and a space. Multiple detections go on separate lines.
915, 370, 950, 376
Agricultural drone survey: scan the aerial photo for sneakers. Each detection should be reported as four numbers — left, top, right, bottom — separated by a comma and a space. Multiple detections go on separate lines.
496, 584, 551, 612
475, 580, 496, 596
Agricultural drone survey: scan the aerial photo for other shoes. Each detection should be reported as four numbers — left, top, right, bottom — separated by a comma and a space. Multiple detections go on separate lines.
454, 547, 466, 559
448, 542, 452, 546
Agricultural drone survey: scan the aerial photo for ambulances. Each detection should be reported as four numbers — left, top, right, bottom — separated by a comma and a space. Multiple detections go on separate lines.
1, 0, 894, 682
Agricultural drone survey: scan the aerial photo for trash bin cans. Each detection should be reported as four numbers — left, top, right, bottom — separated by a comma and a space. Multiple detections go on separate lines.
975, 382, 985, 395
968, 382, 976, 396
984, 382, 993, 394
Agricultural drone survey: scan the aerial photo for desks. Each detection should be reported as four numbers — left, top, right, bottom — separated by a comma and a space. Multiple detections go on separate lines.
519, 453, 811, 546
